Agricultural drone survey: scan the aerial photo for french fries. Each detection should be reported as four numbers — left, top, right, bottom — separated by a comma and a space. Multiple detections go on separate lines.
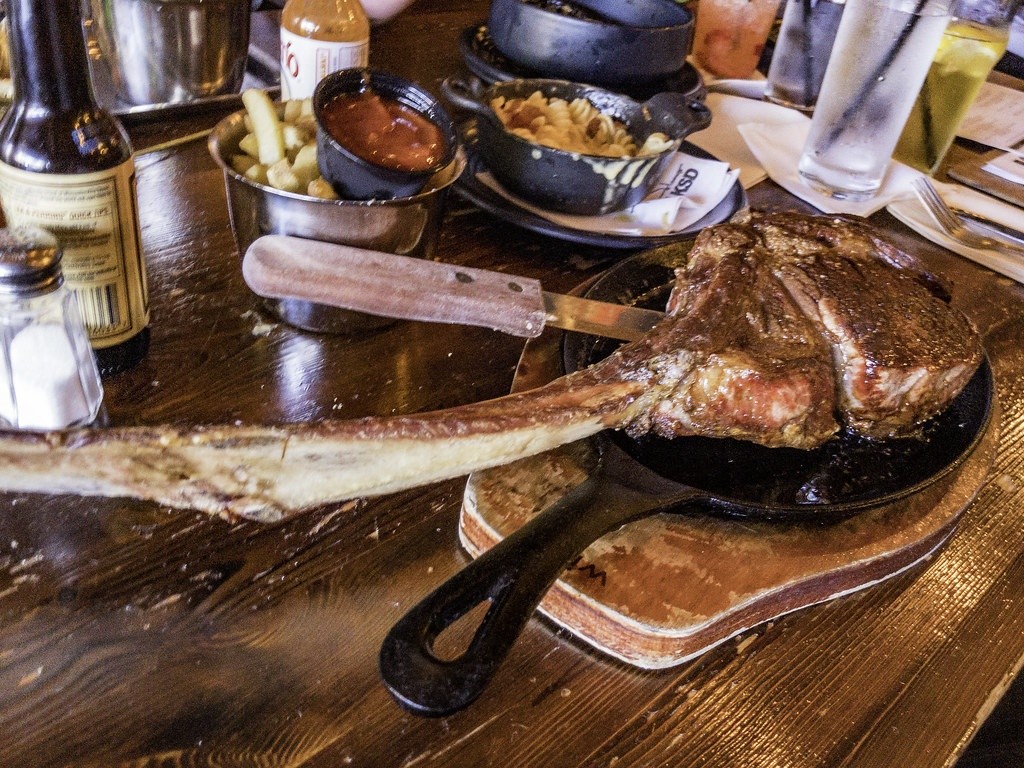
231, 87, 453, 199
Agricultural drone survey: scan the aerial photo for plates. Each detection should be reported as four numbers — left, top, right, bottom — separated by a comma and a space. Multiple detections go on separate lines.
461, 21, 705, 104
452, 118, 747, 248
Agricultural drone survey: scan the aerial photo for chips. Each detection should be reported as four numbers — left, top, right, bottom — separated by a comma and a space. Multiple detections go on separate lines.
492, 91, 680, 158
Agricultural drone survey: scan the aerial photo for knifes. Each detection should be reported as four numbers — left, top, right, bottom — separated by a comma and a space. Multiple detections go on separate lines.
948, 206, 1024, 244
242, 234, 667, 340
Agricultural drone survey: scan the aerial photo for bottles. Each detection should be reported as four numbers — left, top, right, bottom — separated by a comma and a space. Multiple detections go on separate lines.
0, 0, 152, 376
0, 228, 110, 452
280, 0, 371, 105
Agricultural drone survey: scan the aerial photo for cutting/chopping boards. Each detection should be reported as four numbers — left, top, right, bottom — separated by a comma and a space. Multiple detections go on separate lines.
458, 270, 999, 670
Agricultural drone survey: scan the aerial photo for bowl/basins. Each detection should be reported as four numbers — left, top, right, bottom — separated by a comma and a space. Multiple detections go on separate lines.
92, 0, 252, 107
312, 67, 457, 202
491, 0, 695, 87
208, 100, 467, 333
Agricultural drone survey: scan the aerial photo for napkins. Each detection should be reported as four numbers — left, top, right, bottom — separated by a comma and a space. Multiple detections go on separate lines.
681, 79, 927, 221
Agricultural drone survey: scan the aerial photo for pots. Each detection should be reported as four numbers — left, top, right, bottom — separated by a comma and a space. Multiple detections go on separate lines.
443, 75, 712, 216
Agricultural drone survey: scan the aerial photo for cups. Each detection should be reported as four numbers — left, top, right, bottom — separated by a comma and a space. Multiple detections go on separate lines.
891, 0, 1020, 180
691, 0, 781, 85
801, 0, 958, 203
764, 0, 848, 112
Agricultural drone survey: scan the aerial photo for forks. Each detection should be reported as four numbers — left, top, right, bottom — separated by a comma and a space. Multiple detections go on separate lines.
911, 177, 1024, 258
